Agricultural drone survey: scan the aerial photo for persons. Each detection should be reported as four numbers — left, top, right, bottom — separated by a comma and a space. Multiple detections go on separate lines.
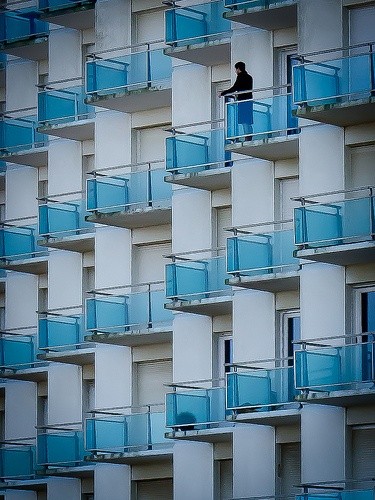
218, 61, 254, 141
